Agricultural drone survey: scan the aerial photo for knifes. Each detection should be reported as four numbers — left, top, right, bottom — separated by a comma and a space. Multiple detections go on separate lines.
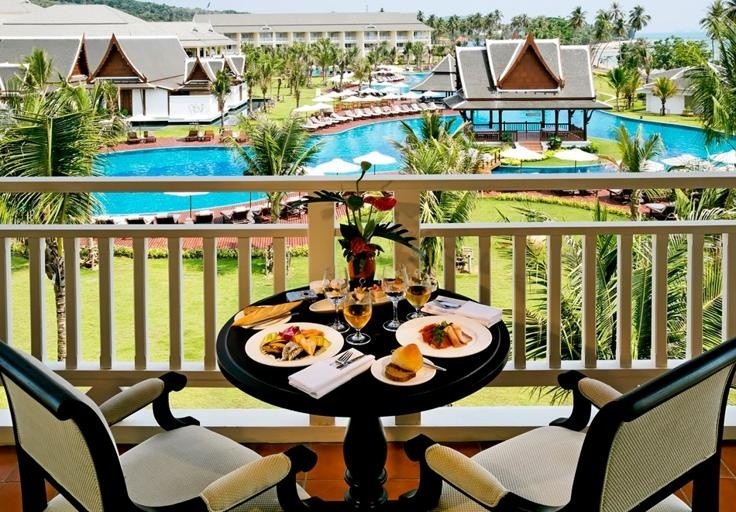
434, 300, 461, 308
337, 354, 367, 370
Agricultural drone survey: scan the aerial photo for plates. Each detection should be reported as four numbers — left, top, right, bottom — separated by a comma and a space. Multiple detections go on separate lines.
243, 322, 344, 367
308, 284, 437, 312
396, 314, 492, 359
234, 305, 292, 330
370, 355, 436, 387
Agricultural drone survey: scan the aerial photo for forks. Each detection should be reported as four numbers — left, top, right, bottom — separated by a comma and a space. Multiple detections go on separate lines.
330, 350, 353, 369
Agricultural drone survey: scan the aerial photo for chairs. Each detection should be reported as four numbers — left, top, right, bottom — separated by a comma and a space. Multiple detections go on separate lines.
0, 340, 330, 511
399, 337, 736, 511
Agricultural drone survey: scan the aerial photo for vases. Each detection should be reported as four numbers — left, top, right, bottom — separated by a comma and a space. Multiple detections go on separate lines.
348, 252, 375, 292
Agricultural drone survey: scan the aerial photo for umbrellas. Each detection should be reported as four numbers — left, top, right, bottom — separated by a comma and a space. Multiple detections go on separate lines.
455, 148, 495, 162
660, 154, 697, 166
554, 148, 599, 173
353, 151, 396, 175
292, 88, 440, 123
709, 149, 736, 164
285, 166, 325, 176
315, 158, 362, 176
616, 159, 664, 172
164, 192, 209, 218
498, 146, 544, 173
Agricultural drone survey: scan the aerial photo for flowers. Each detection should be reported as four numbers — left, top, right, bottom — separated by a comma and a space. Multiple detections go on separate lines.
290, 162, 428, 262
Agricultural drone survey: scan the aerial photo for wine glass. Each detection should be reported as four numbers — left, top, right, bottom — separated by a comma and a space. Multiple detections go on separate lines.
322, 264, 431, 345
